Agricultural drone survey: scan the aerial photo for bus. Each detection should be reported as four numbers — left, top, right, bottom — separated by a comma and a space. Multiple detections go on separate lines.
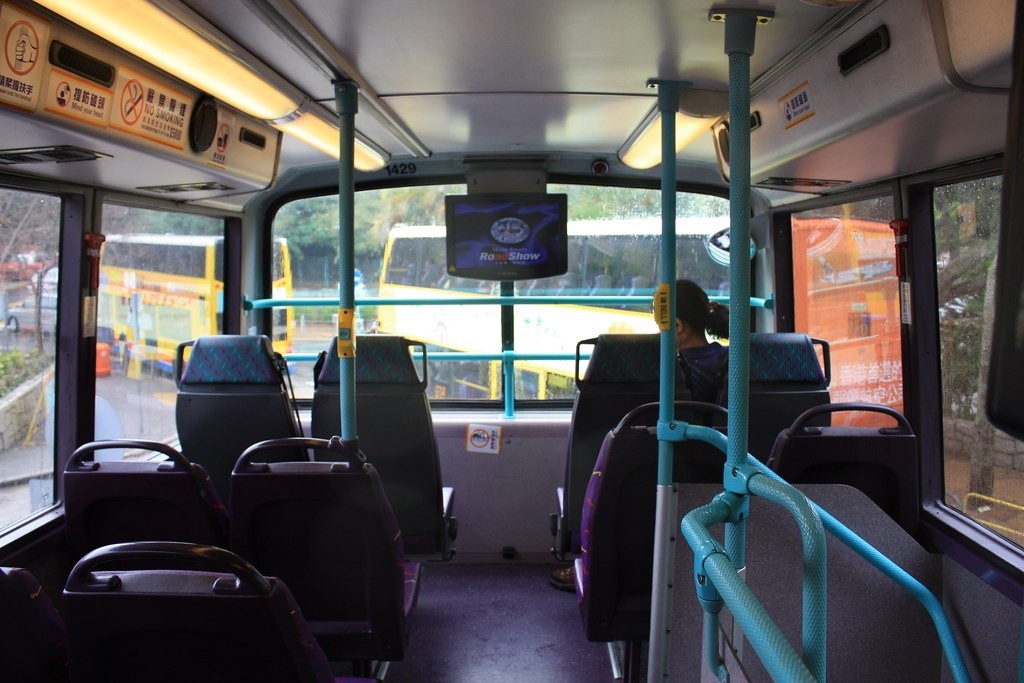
371, 215, 905, 432
98, 233, 296, 377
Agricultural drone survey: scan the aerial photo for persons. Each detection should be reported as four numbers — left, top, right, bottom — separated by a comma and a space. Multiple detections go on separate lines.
549, 279, 729, 592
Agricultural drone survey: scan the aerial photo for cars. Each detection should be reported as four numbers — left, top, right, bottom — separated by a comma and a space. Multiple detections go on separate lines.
1, 252, 51, 280
5, 293, 57, 336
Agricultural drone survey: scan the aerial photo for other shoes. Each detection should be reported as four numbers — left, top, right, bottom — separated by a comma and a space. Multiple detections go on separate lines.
548, 566, 576, 590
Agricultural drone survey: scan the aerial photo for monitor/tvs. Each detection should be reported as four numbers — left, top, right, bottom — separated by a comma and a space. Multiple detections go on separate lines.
445, 193, 568, 281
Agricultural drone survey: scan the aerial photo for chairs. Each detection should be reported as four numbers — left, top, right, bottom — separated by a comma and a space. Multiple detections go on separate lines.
764, 400, 921, 537
0, 565, 73, 682
60, 539, 379, 682
173, 333, 310, 514
61, 437, 243, 571
225, 433, 423, 678
568, 401, 753, 682
309, 334, 459, 564
400, 262, 730, 310
547, 333, 697, 561
704, 331, 831, 485
